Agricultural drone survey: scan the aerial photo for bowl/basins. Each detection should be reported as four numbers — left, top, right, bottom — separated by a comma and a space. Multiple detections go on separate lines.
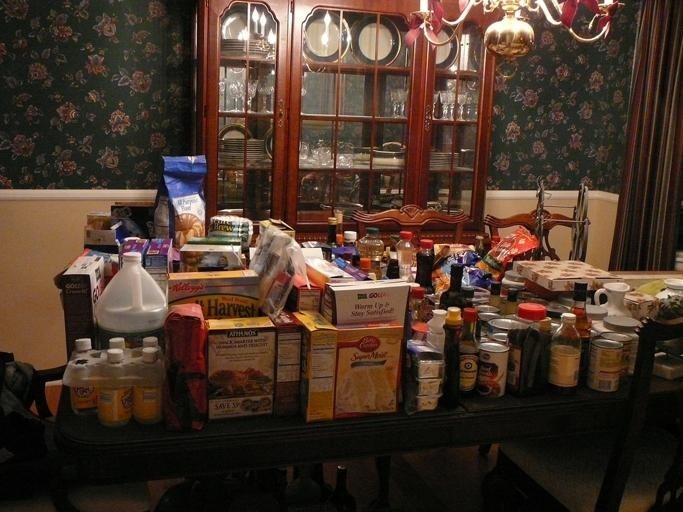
369, 149, 406, 167
350, 146, 378, 160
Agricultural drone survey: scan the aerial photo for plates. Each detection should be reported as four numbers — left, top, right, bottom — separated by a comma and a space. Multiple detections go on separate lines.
264, 127, 274, 160
350, 14, 403, 67
303, 11, 351, 64
218, 123, 253, 139
435, 24, 461, 71
217, 138, 267, 167
429, 151, 460, 169
219, 7, 269, 40
221, 38, 271, 59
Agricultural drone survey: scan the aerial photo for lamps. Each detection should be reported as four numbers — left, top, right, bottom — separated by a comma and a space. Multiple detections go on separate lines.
405, 0, 626, 60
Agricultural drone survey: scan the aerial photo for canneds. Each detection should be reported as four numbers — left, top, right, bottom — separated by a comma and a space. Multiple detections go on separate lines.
487, 318, 528, 346
476, 305, 500, 313
600, 332, 630, 380
478, 341, 510, 398
587, 338, 623, 394
476, 313, 501, 342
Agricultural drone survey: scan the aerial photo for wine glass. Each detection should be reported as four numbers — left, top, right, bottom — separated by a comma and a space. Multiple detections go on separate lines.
463, 96, 473, 121
389, 90, 400, 119
431, 90, 441, 120
241, 78, 258, 114
256, 69, 275, 114
227, 79, 245, 113
394, 88, 409, 119
439, 90, 452, 121
219, 78, 229, 112
456, 94, 467, 122
472, 97, 479, 120
447, 90, 457, 122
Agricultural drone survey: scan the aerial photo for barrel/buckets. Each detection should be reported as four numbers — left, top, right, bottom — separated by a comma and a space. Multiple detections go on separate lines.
88, 251, 169, 350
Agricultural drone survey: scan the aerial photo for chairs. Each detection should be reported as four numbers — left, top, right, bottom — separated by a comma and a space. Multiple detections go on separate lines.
477, 206, 589, 457
1, 351, 80, 512
350, 204, 476, 471
495, 316, 683, 512
145, 462, 356, 512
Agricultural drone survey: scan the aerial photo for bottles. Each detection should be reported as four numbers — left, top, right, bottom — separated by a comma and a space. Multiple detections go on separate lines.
498, 288, 520, 316
154, 195, 170, 240
283, 462, 323, 511
506, 302, 550, 400
537, 316, 554, 354
327, 217, 340, 261
140, 336, 163, 360
403, 287, 427, 370
425, 308, 447, 363
408, 282, 422, 301
592, 316, 642, 376
108, 337, 126, 352
395, 229, 415, 282
439, 262, 465, 318
343, 251, 353, 263
70, 337, 99, 416
460, 285, 482, 343
547, 312, 583, 398
474, 236, 487, 259
96, 348, 132, 429
414, 239, 435, 309
333, 206, 344, 248
336, 230, 360, 270
323, 465, 357, 512
356, 227, 385, 281
385, 258, 400, 280
457, 307, 480, 398
441, 307, 464, 410
488, 282, 503, 310
132, 346, 166, 426
569, 281, 593, 380
359, 257, 371, 274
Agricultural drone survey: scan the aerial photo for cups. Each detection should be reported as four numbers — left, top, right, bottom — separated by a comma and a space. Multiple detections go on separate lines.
298, 139, 354, 169
